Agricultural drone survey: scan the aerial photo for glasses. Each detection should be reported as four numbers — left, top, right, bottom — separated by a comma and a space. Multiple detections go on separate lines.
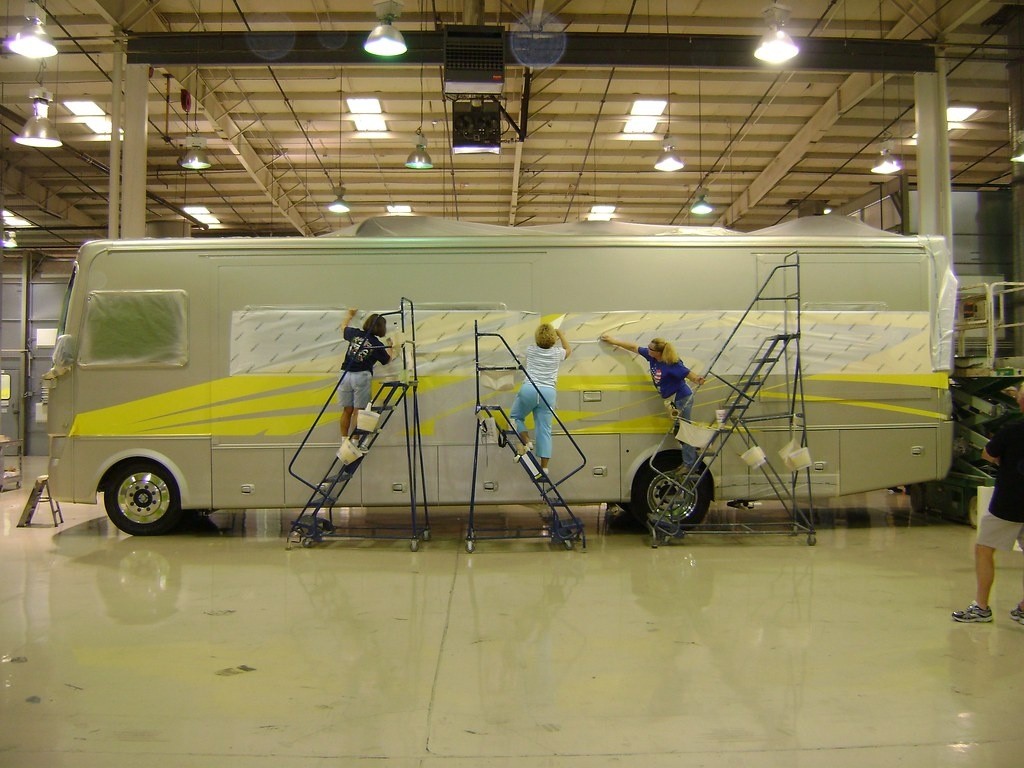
647, 345, 657, 351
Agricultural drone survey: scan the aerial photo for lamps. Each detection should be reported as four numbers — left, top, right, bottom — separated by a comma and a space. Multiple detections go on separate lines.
365, 0, 408, 56
653, 0, 685, 171
405, 62, 433, 168
1010, 131, 1024, 163
8, 0, 59, 58
2, 231, 17, 247
691, 67, 712, 214
328, 66, 350, 212
14, 57, 63, 147
755, 0, 798, 63
870, 1, 901, 176
180, 68, 212, 170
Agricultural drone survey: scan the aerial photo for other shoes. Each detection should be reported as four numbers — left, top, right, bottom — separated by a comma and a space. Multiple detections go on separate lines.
359, 445, 369, 454
675, 464, 695, 477
526, 442, 534, 450
542, 467, 549, 475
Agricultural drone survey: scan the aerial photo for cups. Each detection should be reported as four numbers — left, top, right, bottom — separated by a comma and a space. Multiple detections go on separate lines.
507, 418, 517, 431
715, 410, 725, 427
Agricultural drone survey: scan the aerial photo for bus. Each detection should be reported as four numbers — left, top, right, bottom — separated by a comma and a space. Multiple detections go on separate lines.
45, 234, 957, 538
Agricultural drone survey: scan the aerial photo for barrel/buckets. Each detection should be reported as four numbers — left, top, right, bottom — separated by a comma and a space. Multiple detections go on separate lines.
740, 446, 765, 469
788, 447, 812, 470
357, 403, 380, 433
335, 438, 370, 465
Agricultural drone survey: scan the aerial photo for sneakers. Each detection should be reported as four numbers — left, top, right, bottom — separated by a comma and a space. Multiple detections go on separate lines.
1009, 606, 1024, 624
952, 601, 993, 622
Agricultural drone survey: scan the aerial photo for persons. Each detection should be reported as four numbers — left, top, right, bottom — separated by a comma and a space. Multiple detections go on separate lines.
509, 323, 571, 482
601, 335, 707, 476
951, 380, 1024, 625
337, 307, 392, 453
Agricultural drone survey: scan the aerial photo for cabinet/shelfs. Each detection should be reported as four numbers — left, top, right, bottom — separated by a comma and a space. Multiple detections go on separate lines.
0, 439, 22, 492
